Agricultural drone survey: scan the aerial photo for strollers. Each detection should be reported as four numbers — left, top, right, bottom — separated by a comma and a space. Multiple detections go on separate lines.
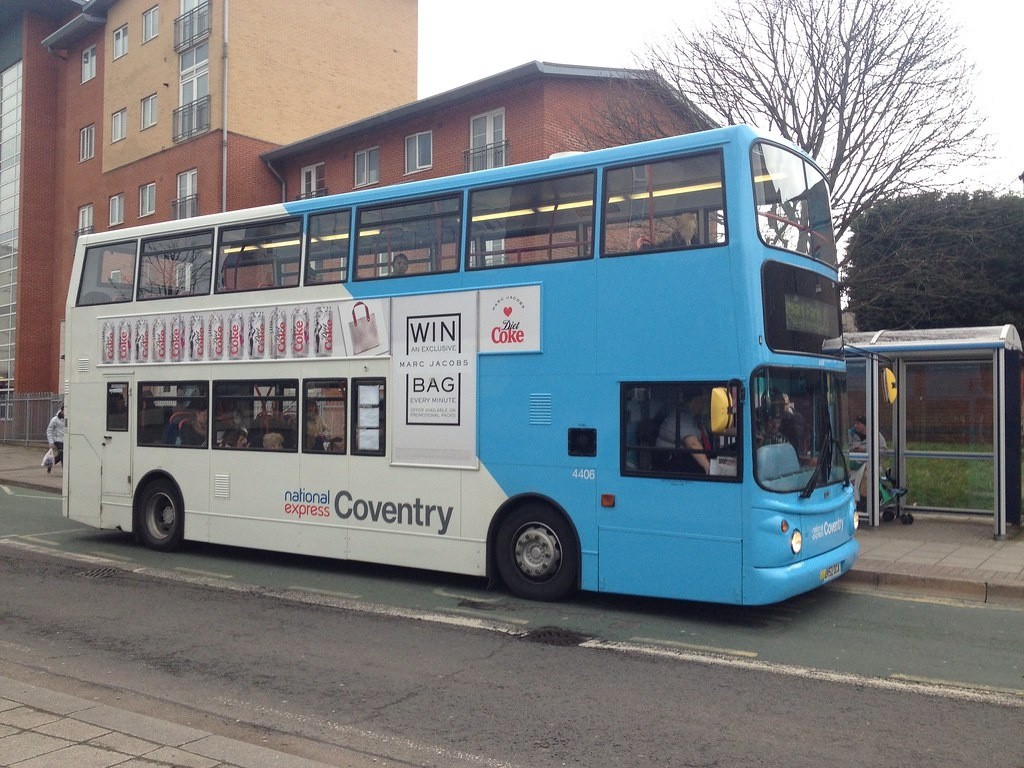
851, 460, 914, 524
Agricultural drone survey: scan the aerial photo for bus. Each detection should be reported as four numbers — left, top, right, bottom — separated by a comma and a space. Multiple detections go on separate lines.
60, 122, 859, 609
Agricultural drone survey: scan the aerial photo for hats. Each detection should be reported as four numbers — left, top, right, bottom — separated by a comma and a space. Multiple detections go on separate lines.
853, 415, 866, 425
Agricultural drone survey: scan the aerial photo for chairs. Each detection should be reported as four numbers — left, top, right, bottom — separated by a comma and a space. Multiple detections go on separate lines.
106, 391, 296, 451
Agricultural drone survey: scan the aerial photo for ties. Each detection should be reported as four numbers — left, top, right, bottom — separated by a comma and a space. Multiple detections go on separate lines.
697, 422, 711, 450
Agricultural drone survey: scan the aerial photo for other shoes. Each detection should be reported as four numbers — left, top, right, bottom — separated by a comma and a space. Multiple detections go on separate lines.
47, 467, 51, 473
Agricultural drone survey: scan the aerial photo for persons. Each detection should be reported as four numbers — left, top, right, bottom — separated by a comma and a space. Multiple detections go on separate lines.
387, 253, 409, 277
45, 405, 65, 475
624, 384, 821, 476
106, 381, 342, 452
664, 214, 701, 250
847, 413, 886, 512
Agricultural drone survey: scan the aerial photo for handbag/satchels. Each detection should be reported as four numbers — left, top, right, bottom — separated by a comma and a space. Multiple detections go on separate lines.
41, 448, 55, 467
348, 302, 379, 355
